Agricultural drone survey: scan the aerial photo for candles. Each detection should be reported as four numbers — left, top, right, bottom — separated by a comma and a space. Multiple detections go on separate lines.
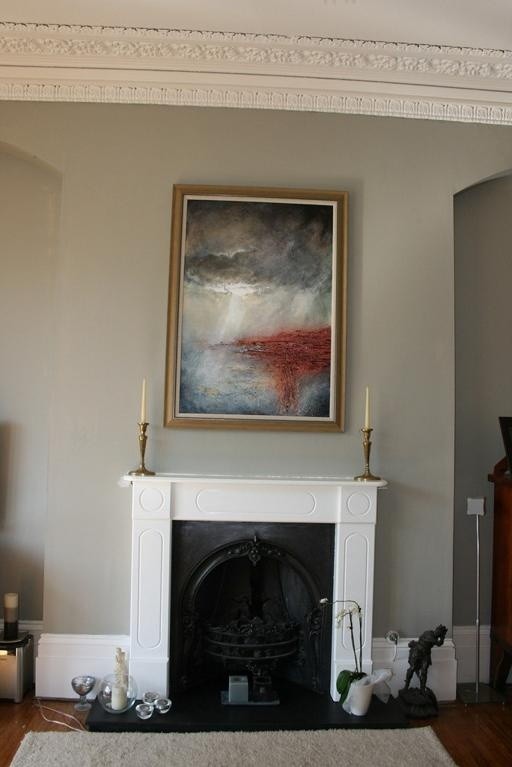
141, 379, 147, 424
364, 387, 370, 430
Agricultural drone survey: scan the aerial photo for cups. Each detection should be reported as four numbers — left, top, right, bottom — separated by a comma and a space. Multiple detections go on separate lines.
153, 696, 172, 713
143, 686, 160, 707
135, 703, 155, 719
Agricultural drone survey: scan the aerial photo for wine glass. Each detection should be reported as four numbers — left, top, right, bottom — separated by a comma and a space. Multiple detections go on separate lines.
70, 676, 96, 712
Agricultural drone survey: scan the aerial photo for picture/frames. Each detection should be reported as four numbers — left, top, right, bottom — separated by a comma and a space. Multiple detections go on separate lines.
164, 183, 349, 431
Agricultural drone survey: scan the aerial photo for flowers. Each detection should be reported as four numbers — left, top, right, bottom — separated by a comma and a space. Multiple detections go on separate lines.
319, 598, 387, 695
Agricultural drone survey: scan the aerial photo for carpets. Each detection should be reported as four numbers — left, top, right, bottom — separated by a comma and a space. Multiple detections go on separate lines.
8, 728, 458, 767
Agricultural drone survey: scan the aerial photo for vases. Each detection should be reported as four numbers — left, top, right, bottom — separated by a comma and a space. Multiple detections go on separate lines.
337, 675, 382, 714
